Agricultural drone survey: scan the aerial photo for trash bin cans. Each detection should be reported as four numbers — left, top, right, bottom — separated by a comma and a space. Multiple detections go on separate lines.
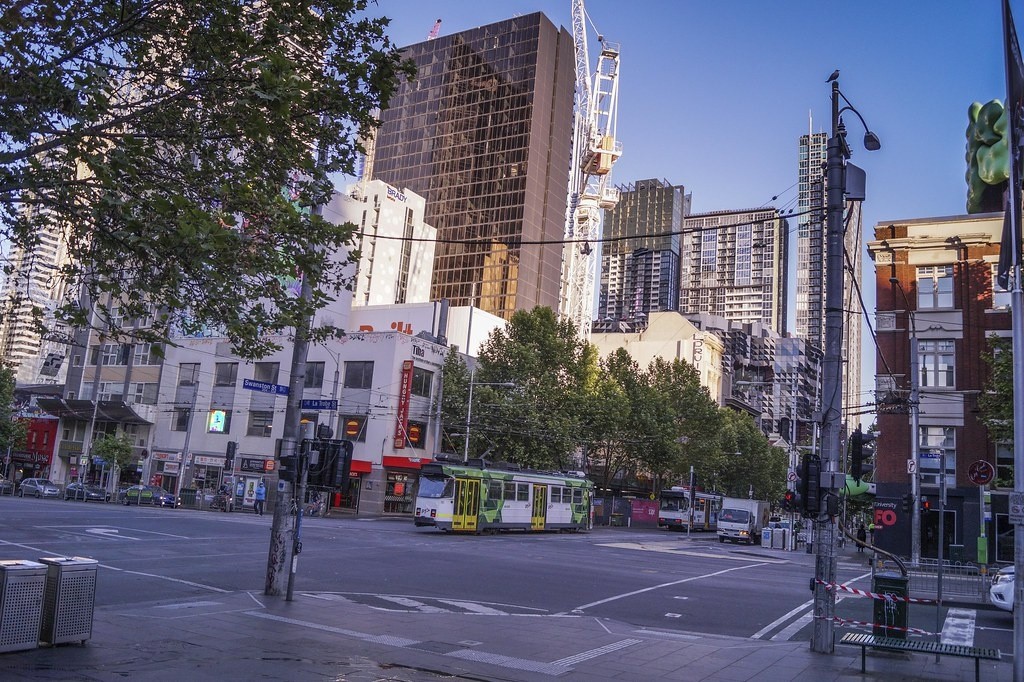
784, 529, 797, 550
873, 573, 909, 653
0, 560, 49, 652
772, 529, 785, 549
620, 514, 623, 526
762, 527, 773, 549
38, 556, 100, 644
611, 513, 619, 526
950, 544, 965, 569
180, 488, 198, 506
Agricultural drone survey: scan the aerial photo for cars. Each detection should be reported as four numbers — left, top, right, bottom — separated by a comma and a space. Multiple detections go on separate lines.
118, 485, 181, 508
0, 473, 63, 498
64, 482, 111, 503
988, 565, 1016, 613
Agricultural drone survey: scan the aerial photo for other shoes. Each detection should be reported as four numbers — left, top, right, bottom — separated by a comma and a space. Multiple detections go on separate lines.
255, 513, 259, 517
259, 515, 264, 517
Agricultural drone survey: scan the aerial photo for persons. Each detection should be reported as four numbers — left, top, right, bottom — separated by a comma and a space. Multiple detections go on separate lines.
856, 525, 866, 552
196, 487, 202, 509
313, 491, 321, 506
869, 521, 875, 543
220, 482, 229, 494
150, 479, 155, 485
775, 522, 781, 528
254, 482, 265, 516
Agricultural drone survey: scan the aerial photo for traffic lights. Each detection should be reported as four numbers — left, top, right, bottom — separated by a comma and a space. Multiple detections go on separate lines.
796, 454, 820, 517
225, 440, 236, 461
902, 492, 912, 512
851, 423, 875, 479
785, 491, 795, 504
922, 500, 931, 510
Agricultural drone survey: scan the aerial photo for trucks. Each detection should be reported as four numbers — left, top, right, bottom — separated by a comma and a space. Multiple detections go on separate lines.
716, 496, 771, 545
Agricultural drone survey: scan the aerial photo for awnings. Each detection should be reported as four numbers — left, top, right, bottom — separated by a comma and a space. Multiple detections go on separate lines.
350, 416, 372, 473
383, 412, 459, 469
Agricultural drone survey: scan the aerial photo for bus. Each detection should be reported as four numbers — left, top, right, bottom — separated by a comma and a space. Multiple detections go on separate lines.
413, 453, 595, 533
658, 486, 722, 531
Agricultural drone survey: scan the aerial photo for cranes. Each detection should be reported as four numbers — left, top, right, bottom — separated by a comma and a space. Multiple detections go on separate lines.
556, 1, 621, 385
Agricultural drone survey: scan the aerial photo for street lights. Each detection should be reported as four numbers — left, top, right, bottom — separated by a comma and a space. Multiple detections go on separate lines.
809, 82, 880, 651
908, 390, 982, 567
464, 370, 515, 462
